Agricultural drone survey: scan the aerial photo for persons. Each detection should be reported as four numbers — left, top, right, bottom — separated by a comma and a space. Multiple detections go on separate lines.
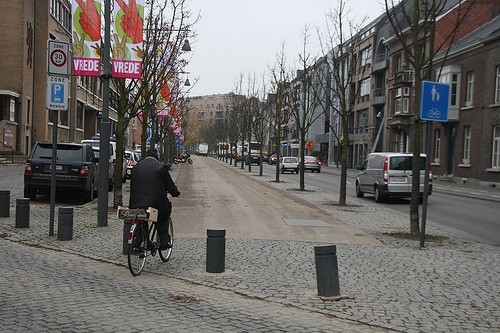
129, 149, 181, 251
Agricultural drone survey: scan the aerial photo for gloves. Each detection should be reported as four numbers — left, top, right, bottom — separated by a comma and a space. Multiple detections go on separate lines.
172, 190, 180, 197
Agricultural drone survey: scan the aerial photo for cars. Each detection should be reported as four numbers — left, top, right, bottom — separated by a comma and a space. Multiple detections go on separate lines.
279, 157, 299, 174
298, 155, 321, 173
267, 154, 277, 165
124, 149, 141, 176
245, 154, 260, 166
195, 148, 199, 155
262, 155, 269, 162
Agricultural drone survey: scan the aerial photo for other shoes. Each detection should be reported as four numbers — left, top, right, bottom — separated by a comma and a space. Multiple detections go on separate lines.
159, 243, 173, 250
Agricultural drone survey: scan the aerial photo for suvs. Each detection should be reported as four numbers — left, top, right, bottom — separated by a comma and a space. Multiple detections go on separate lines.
81, 137, 130, 191
23, 141, 99, 202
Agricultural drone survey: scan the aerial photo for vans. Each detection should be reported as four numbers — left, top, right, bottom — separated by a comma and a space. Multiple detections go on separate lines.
355, 152, 433, 202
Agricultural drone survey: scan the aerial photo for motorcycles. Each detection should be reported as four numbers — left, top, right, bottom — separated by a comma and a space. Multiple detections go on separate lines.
174, 153, 193, 164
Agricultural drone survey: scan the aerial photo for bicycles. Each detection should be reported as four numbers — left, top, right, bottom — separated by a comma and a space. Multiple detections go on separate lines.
122, 192, 180, 277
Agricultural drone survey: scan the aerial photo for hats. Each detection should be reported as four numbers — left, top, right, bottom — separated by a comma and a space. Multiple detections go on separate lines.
147, 148, 158, 158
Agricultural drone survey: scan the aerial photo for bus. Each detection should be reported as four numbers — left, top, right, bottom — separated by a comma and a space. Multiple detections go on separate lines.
199, 143, 209, 156
216, 142, 247, 161
246, 142, 262, 156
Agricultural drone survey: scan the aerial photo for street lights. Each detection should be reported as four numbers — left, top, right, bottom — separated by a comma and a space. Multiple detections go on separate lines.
140, 28, 192, 138
